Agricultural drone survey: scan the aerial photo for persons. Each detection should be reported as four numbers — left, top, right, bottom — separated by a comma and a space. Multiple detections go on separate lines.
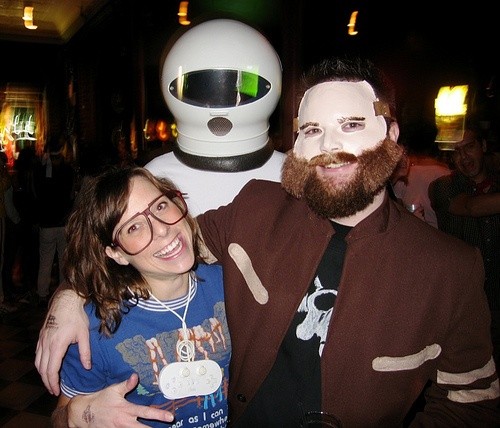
50, 167, 229, 428
141, 17, 286, 219
391, 118, 500, 372
49, 50, 499, 428
0, 139, 80, 313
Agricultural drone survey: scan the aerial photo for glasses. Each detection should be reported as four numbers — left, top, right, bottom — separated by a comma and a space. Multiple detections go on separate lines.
110, 189, 189, 257
